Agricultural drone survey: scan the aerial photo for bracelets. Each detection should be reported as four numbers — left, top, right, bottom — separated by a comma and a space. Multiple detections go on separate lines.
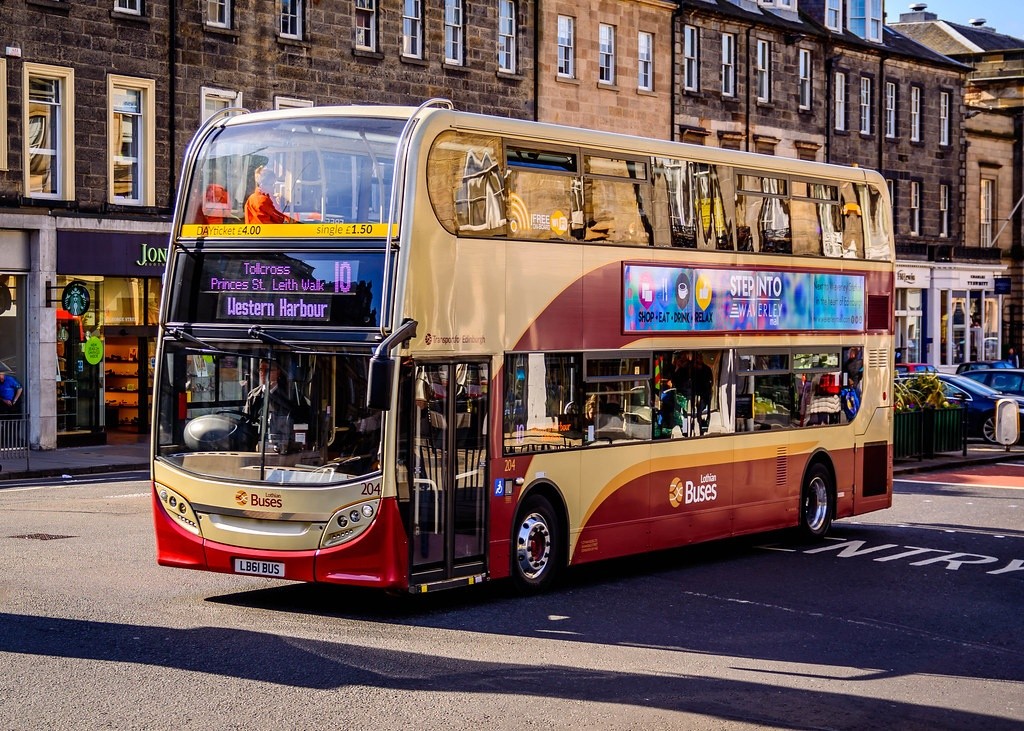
13, 400, 17, 402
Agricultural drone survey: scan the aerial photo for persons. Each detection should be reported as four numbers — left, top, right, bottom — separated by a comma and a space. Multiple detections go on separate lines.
244, 166, 302, 224
0, 372, 23, 420
240, 357, 290, 422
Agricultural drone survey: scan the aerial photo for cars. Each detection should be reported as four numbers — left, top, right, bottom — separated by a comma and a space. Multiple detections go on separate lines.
961, 368, 1024, 399
956, 359, 1018, 373
894, 371, 1024, 446
894, 362, 940, 373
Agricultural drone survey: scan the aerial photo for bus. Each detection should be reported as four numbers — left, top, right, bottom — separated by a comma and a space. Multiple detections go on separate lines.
148, 99, 899, 596
